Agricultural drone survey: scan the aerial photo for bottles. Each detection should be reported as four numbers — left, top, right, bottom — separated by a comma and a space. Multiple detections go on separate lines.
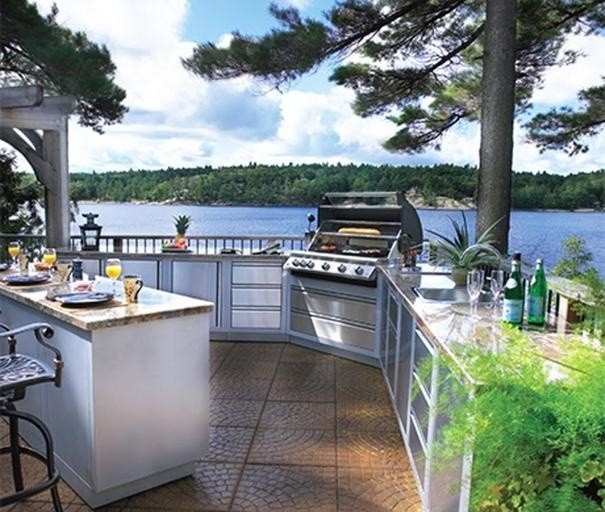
72, 259, 82, 281
422, 239, 430, 260
503, 252, 525, 327
529, 256, 550, 323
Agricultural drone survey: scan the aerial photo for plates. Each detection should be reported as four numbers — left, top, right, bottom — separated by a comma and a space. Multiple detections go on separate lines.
2, 273, 50, 283
52, 293, 113, 306
159, 247, 193, 253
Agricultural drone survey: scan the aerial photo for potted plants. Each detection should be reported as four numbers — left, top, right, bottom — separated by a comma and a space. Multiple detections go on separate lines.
415, 209, 508, 286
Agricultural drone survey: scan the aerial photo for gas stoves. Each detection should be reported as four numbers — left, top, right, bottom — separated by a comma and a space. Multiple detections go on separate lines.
284, 241, 389, 281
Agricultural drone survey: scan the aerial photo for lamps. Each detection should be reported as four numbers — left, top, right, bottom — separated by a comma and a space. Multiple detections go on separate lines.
78, 212, 103, 251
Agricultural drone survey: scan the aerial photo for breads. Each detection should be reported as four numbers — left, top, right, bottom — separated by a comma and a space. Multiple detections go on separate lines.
337, 226, 382, 236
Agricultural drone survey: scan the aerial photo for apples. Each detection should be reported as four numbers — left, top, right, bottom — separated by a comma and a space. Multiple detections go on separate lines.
163, 239, 187, 248
320, 244, 336, 251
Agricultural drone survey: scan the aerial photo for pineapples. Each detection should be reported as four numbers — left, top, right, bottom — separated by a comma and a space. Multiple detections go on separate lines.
172, 213, 193, 244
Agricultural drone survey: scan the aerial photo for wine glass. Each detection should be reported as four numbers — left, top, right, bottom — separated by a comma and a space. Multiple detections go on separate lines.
466, 269, 483, 322
487, 270, 505, 313
8, 242, 20, 270
44, 246, 56, 271
106, 258, 122, 298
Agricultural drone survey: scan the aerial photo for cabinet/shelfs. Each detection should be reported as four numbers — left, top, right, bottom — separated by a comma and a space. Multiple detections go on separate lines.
379, 278, 472, 512
69, 258, 282, 331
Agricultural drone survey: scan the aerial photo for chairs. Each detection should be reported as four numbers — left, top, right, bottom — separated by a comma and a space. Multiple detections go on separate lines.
0, 309, 65, 512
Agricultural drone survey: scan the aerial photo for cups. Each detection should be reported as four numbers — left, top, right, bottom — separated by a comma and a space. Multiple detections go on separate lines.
400, 248, 415, 270
121, 274, 143, 303
